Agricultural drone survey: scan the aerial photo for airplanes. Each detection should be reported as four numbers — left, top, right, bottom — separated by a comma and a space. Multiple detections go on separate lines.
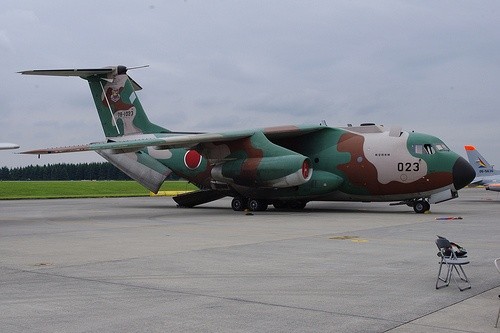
15, 65, 477, 214
464, 145, 500, 193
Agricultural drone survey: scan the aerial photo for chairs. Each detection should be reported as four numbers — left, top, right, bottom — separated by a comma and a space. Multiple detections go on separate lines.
494, 257, 500, 329
434, 239, 471, 291
436, 235, 468, 282
